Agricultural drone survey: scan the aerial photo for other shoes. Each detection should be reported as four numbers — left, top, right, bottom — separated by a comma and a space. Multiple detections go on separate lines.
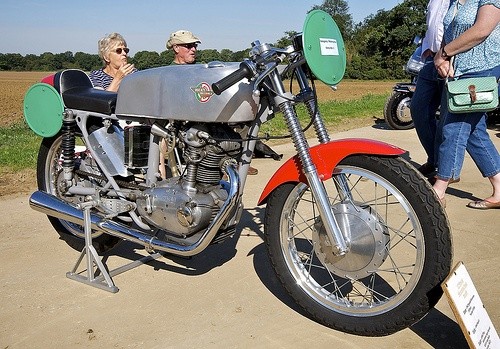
440, 198, 448, 209
468, 198, 500, 210
417, 162, 460, 186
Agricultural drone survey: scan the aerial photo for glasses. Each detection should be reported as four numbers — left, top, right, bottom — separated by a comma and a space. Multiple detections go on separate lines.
179, 43, 198, 49
110, 48, 130, 54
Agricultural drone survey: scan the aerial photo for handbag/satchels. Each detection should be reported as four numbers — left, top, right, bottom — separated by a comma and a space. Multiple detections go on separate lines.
445, 76, 499, 114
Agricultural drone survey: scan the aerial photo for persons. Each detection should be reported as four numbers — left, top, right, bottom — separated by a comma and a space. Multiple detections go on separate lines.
434, 0, 500, 210
410, 0, 461, 186
166, 30, 202, 65
88, 33, 140, 92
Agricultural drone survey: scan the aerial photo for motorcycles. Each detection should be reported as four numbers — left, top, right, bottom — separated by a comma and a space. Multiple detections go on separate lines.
24, 9, 454, 338
383, 27, 500, 130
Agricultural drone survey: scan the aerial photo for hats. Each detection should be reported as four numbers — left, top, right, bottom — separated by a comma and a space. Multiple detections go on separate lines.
166, 30, 201, 49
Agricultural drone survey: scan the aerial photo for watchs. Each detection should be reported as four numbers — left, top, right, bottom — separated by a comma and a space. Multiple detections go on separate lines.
441, 46, 449, 60
